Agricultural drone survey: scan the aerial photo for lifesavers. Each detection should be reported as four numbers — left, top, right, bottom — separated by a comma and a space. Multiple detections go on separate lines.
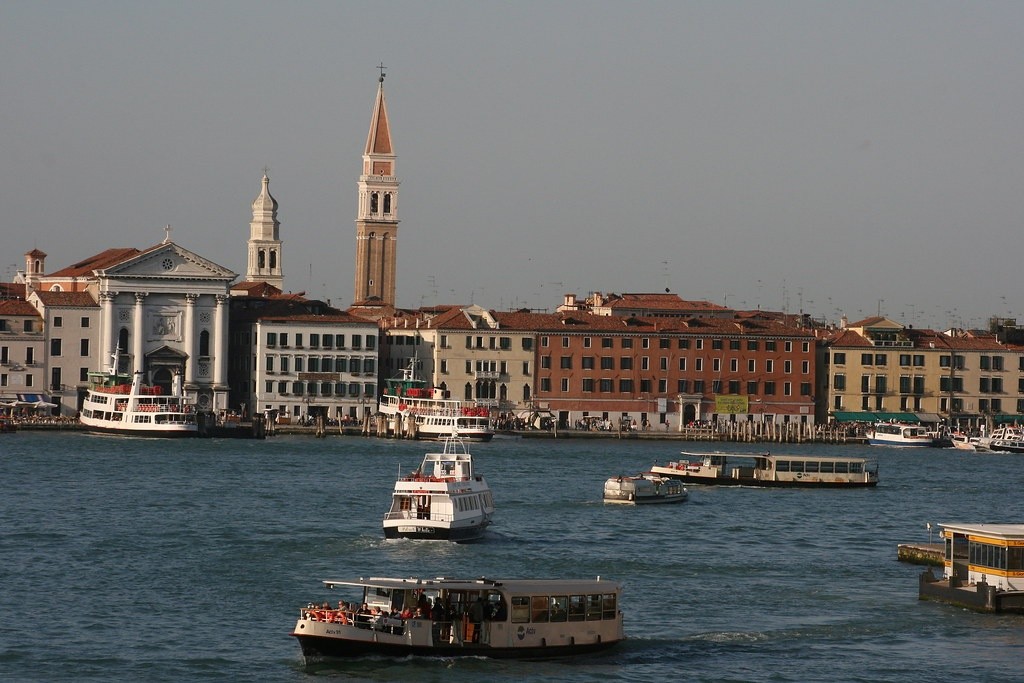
304, 610, 347, 624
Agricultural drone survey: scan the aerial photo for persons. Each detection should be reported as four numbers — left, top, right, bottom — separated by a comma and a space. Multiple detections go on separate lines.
815, 423, 1024, 437
296, 410, 392, 427
689, 419, 797, 433
492, 416, 670, 433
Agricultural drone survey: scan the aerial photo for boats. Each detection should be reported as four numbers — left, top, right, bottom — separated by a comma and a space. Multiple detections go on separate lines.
863, 419, 1024, 456
381, 434, 494, 546
379, 350, 496, 442
642, 452, 881, 488
79, 338, 201, 437
601, 474, 689, 506
287, 575, 626, 666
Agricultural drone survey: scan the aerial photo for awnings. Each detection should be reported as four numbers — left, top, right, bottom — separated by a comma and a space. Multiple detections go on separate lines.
830, 410, 941, 424
